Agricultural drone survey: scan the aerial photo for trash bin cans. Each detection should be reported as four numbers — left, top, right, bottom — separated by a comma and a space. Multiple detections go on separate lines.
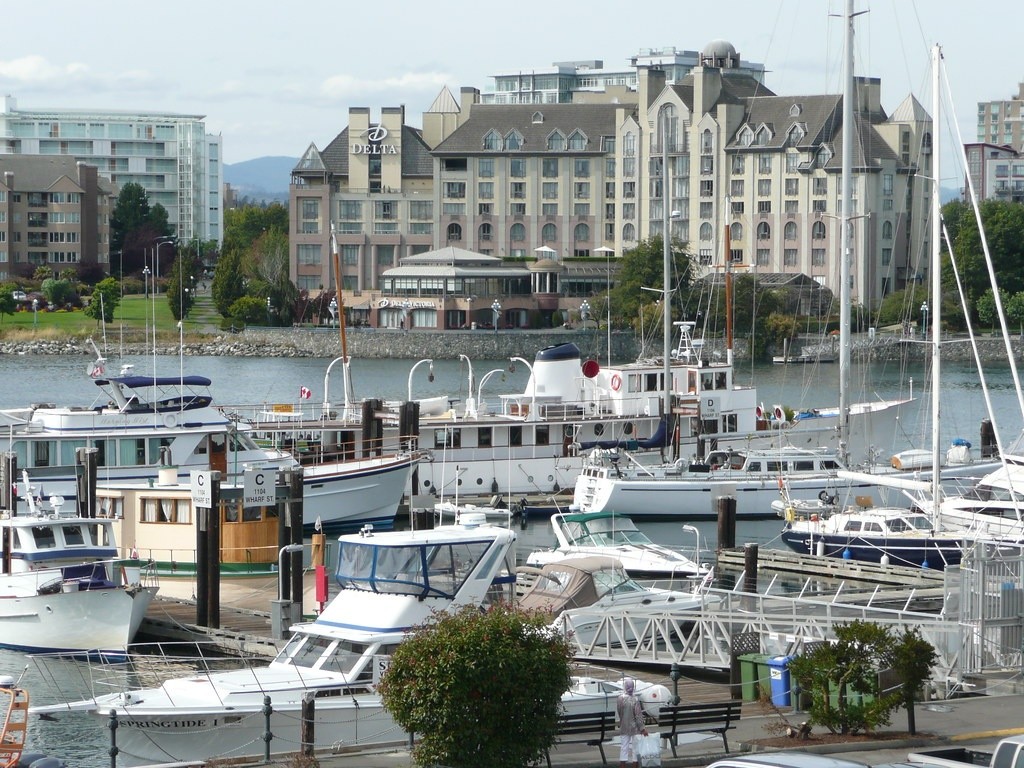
790, 661, 878, 716
768, 655, 794, 706
737, 653, 762, 700
753, 654, 774, 699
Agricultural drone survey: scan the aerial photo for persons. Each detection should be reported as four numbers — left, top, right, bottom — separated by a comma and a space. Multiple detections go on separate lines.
717, 373, 725, 387
306, 434, 314, 451
400, 317, 404, 330
617, 679, 649, 768
296, 434, 308, 451
704, 379, 712, 389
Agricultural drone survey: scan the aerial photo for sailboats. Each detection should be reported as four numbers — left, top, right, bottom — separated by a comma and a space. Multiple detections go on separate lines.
256, 106, 917, 495
558, 0, 1024, 566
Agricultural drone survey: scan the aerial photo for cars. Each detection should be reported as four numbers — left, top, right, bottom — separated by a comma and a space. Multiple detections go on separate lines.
12, 291, 26, 299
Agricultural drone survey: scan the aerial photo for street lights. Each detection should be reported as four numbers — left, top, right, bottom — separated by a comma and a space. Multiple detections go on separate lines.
330, 298, 338, 330
580, 300, 590, 330
920, 301, 929, 335
142, 266, 151, 298
157, 241, 173, 294
491, 299, 501, 332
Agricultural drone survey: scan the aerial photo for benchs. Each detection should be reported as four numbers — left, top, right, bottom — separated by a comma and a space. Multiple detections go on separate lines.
539, 699, 743, 767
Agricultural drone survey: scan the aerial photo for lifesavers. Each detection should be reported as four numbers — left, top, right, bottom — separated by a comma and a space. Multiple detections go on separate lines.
818, 490, 828, 500
163, 413, 178, 429
610, 375, 621, 390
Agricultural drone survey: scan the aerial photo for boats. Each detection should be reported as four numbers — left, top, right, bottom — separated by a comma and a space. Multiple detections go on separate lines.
0, 221, 445, 663
516, 508, 708, 644
28, 493, 669, 768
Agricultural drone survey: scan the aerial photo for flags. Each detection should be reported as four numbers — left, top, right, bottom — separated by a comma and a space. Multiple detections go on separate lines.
300, 386, 312, 400
91, 366, 104, 378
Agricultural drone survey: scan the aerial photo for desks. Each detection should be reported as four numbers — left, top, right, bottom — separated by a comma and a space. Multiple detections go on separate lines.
262, 412, 304, 429
498, 394, 563, 417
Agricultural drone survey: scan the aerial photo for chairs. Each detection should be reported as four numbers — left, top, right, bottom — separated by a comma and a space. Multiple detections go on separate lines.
475, 402, 488, 418
449, 403, 467, 422
